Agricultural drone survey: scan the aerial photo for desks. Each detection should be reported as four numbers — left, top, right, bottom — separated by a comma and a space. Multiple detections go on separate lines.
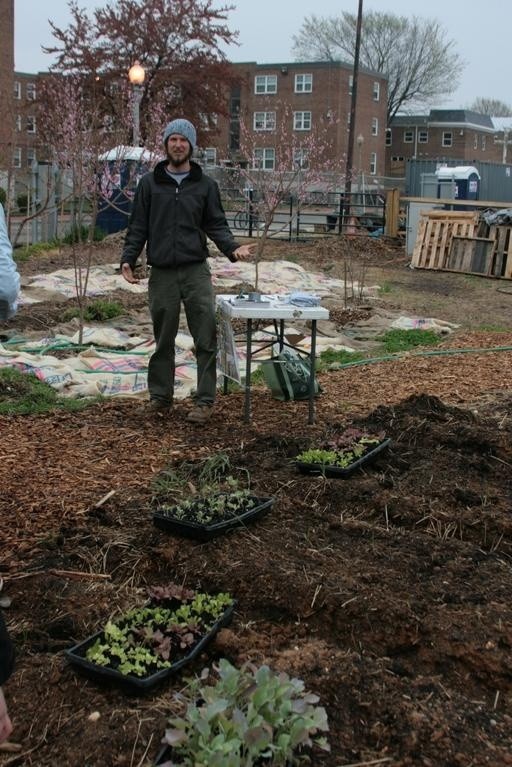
215, 287, 330, 429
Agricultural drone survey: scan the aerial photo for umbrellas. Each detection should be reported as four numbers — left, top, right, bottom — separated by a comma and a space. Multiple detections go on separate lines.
98, 142, 159, 163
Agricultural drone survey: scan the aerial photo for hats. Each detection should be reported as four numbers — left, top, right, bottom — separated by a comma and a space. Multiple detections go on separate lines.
163, 118, 196, 151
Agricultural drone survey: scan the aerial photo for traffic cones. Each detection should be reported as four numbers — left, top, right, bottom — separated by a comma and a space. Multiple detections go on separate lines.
342, 216, 358, 242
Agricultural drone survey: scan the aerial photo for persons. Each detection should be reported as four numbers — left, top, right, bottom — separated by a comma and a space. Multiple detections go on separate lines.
0, 204, 22, 323
120, 118, 260, 424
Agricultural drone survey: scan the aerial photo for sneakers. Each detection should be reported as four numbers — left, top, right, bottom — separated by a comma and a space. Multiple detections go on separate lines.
132, 399, 172, 416
184, 402, 215, 424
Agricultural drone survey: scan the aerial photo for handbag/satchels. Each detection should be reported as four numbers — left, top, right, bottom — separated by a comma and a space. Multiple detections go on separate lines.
263, 346, 322, 401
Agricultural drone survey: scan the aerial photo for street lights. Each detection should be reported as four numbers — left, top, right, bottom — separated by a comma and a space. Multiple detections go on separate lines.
357, 131, 366, 210
128, 59, 147, 149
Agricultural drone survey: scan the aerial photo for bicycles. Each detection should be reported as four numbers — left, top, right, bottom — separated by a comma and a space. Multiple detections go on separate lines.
233, 204, 274, 230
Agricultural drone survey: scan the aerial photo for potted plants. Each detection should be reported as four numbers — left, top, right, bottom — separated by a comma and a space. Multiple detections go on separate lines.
64, 588, 236, 696
296, 424, 395, 477
150, 477, 275, 541
